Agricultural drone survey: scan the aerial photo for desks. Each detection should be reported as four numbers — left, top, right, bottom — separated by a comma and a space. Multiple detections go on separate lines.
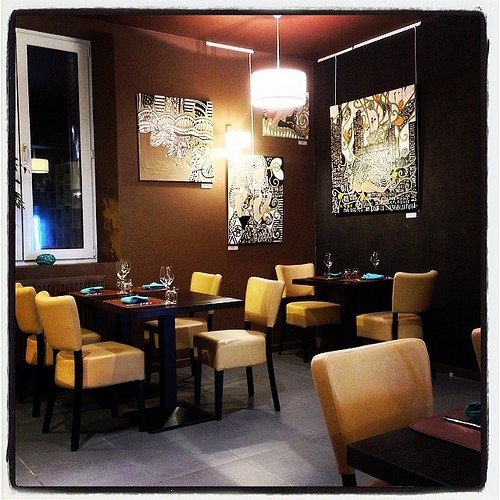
346, 408, 482, 491
67, 287, 244, 434
291, 274, 394, 349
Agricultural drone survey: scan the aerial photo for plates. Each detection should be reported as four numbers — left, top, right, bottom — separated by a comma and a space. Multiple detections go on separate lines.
102, 297, 171, 308
67, 289, 118, 297
132, 287, 179, 293
442, 408, 482, 430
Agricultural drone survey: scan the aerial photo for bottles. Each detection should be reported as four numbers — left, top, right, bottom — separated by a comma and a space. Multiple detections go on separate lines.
164, 285, 178, 304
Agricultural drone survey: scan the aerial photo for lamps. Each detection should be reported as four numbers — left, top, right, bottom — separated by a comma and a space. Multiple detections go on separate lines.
251, 15, 307, 110
31, 147, 49, 174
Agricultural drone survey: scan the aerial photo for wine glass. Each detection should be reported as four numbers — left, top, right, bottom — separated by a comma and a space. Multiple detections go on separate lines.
370, 252, 380, 274
160, 265, 175, 303
323, 252, 334, 280
116, 259, 132, 294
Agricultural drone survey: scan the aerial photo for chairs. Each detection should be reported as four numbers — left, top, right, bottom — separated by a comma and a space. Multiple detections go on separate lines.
35, 291, 157, 451
311, 338, 435, 486
16, 282, 101, 418
193, 277, 285, 421
357, 270, 437, 344
144, 272, 222, 374
275, 263, 342, 363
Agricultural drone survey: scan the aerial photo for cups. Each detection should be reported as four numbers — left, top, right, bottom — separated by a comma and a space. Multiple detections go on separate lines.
117, 279, 132, 295
